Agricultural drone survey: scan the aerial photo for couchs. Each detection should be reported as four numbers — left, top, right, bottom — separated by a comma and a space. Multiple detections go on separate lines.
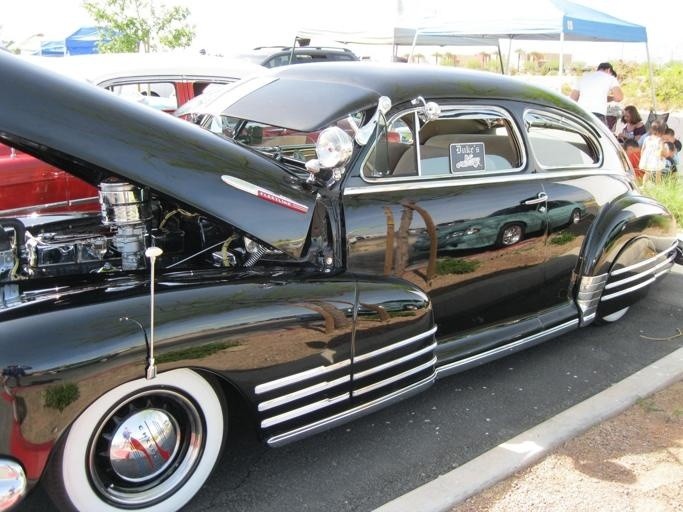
424, 134, 588, 168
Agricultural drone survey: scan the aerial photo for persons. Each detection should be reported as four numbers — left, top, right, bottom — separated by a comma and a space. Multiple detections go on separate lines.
568, 60, 623, 125
637, 118, 668, 188
672, 138, 682, 153
620, 138, 644, 178
661, 141, 677, 173
606, 103, 622, 131
663, 127, 675, 145
616, 104, 645, 143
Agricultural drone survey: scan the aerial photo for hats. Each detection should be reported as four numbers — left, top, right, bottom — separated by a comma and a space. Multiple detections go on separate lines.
597, 63, 617, 77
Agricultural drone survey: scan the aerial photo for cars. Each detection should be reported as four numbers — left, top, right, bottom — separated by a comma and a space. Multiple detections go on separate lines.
338, 296, 428, 316
1, 49, 407, 221
0, 61, 682, 510
411, 195, 587, 254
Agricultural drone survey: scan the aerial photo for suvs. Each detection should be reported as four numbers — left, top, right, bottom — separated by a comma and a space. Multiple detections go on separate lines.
247, 44, 359, 72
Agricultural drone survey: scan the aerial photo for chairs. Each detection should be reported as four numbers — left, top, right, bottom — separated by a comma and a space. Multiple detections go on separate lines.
393, 146, 450, 177
366, 143, 410, 177
